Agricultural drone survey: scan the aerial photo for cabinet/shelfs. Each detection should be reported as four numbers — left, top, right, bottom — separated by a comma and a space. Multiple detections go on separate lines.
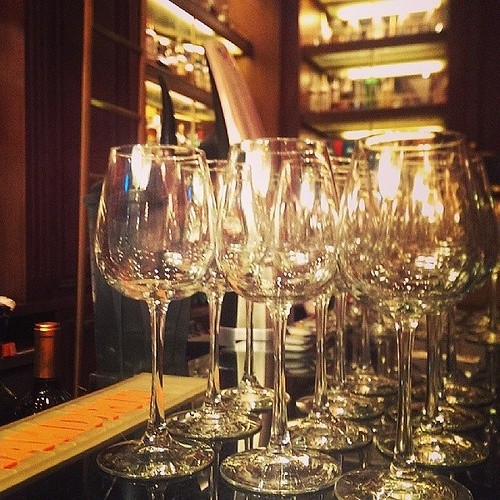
23, 0, 500, 400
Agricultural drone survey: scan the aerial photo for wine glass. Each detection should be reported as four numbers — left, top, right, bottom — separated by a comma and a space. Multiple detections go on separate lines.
336, 128, 473, 500
215, 139, 339, 494
95, 144, 215, 479
166, 127, 494, 466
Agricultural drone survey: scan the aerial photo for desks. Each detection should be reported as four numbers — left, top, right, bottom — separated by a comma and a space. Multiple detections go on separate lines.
23, 315, 500, 500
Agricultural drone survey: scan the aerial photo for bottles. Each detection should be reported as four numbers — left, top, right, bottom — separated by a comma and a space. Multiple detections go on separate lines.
15, 322, 73, 419
310, 72, 445, 108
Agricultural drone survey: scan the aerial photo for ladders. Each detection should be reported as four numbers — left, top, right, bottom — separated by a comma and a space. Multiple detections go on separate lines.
76, 1, 146, 384
